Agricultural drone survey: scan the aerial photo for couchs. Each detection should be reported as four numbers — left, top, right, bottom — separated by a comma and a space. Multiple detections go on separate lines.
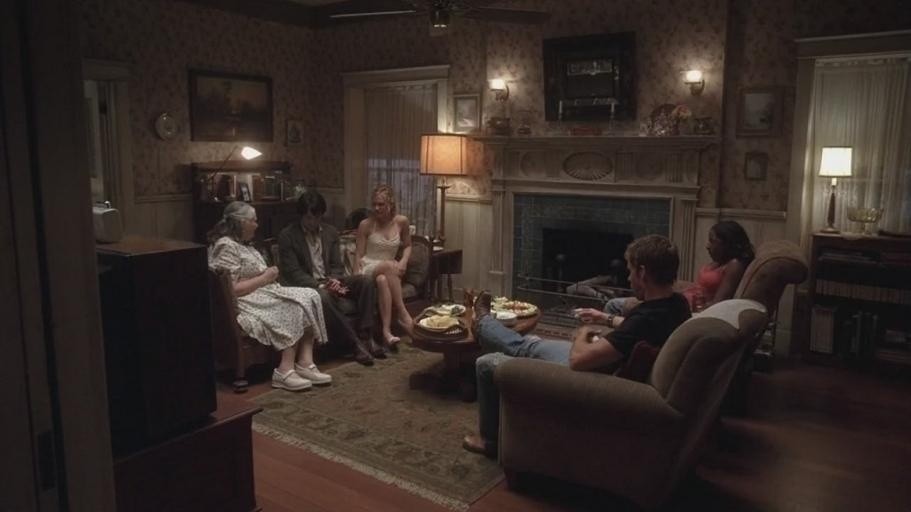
624, 235, 812, 358
488, 296, 772, 510
194, 228, 433, 392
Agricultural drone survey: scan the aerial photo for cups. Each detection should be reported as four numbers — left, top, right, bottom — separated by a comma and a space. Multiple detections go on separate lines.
574, 307, 584, 318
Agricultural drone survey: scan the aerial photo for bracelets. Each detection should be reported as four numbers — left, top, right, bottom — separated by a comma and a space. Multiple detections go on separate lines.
607, 314, 616, 327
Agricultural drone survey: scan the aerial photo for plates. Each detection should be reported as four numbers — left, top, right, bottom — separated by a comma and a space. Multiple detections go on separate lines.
419, 301, 537, 333
650, 104, 680, 136
154, 113, 183, 140
425, 236, 444, 252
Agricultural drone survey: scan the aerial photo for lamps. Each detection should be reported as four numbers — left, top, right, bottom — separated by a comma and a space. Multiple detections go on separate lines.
204, 144, 264, 184
416, 133, 470, 246
489, 76, 511, 101
429, 12, 451, 28
679, 69, 708, 95
819, 143, 856, 234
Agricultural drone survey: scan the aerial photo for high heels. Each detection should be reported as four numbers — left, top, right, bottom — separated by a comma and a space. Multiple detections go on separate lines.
397, 317, 422, 341
381, 329, 401, 348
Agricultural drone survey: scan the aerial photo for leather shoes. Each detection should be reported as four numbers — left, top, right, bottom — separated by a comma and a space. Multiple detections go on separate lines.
470, 288, 493, 338
293, 361, 332, 385
349, 339, 373, 363
364, 337, 386, 357
462, 432, 499, 460
270, 367, 312, 392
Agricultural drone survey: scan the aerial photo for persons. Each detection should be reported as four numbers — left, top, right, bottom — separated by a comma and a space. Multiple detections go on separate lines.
603, 220, 755, 313
352, 184, 414, 347
463, 234, 692, 458
205, 200, 333, 391
276, 189, 387, 366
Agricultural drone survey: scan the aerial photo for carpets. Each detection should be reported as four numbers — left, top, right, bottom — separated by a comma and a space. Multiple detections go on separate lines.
242, 328, 586, 512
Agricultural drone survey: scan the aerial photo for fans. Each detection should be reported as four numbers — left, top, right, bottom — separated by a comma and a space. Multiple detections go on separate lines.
328, 0, 552, 30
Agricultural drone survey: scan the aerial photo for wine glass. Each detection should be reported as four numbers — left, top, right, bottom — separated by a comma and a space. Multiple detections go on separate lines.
848, 204, 884, 235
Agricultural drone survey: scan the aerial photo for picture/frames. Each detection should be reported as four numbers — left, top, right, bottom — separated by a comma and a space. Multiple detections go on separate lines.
454, 93, 484, 135
744, 150, 769, 180
187, 69, 275, 145
282, 118, 307, 147
734, 86, 787, 138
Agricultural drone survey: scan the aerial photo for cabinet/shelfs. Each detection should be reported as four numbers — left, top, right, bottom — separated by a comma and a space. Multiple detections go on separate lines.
109, 390, 271, 512
804, 232, 911, 369
192, 157, 306, 248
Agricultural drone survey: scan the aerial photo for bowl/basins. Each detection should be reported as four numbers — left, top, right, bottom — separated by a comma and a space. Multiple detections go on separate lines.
486, 117, 510, 129
